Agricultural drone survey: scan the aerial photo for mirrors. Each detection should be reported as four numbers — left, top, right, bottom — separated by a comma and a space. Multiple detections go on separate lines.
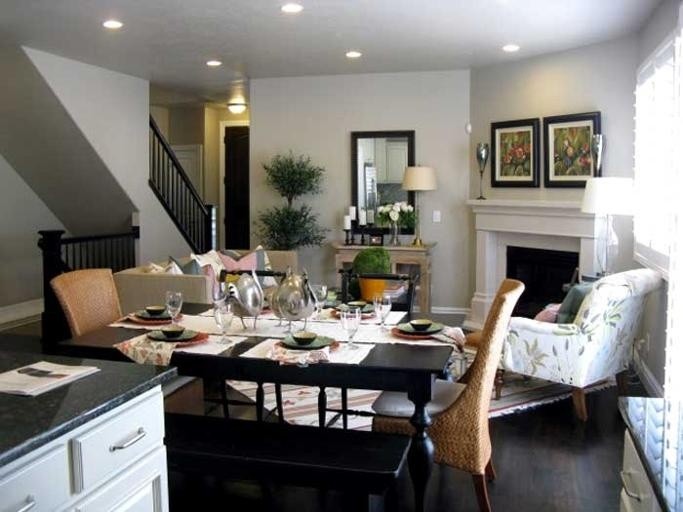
348, 126, 419, 237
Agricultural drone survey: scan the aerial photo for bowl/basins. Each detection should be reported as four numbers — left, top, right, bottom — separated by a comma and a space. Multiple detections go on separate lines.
408, 318, 431, 331
347, 302, 366, 313
160, 325, 185, 338
291, 330, 316, 345
145, 305, 166, 316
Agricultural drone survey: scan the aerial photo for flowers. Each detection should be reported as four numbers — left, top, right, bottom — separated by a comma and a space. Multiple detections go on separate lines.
373, 202, 414, 231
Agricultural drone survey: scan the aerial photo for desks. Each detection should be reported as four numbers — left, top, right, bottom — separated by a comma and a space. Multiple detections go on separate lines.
328, 238, 438, 313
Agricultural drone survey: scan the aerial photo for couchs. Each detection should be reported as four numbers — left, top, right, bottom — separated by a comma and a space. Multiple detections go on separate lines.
109, 245, 301, 312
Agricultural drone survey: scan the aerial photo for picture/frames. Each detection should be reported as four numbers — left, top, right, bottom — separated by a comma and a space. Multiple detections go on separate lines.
485, 115, 543, 192
540, 109, 604, 192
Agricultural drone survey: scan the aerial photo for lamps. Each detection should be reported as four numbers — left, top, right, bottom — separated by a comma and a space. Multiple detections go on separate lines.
395, 162, 436, 247
224, 101, 247, 117
574, 172, 637, 277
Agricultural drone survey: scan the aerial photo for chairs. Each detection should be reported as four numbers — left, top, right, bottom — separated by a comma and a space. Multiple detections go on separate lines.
47, 266, 207, 414
313, 266, 417, 432
492, 259, 662, 433
368, 274, 529, 510
201, 268, 291, 425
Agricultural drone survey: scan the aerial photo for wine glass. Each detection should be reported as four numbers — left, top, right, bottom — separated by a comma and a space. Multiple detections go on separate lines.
475, 142, 488, 200
340, 305, 360, 350
590, 133, 602, 177
216, 312, 233, 344
374, 295, 391, 334
164, 291, 183, 323
310, 285, 327, 320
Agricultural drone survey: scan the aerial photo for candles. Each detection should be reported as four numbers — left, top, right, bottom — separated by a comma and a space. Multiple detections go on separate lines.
365, 208, 375, 224
341, 213, 354, 229
358, 209, 367, 226
347, 202, 356, 219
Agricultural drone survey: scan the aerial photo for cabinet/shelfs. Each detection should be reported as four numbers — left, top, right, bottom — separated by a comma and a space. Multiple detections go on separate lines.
0, 382, 170, 511
611, 391, 665, 510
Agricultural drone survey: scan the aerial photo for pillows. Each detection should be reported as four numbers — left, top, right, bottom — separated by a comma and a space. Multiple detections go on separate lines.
555, 277, 593, 326
533, 298, 561, 324
141, 242, 276, 291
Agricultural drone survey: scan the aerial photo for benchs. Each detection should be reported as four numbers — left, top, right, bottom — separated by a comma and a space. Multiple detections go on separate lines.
160, 408, 413, 510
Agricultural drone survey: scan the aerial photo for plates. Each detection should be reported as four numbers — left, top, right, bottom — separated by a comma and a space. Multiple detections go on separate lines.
283, 335, 335, 349
330, 306, 375, 319
147, 330, 198, 340
274, 339, 339, 354
135, 310, 172, 319
175, 332, 208, 346
396, 322, 443, 335
391, 327, 434, 338
127, 313, 183, 323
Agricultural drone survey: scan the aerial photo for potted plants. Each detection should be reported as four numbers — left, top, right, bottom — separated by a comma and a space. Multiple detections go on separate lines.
245, 149, 335, 276
351, 247, 394, 301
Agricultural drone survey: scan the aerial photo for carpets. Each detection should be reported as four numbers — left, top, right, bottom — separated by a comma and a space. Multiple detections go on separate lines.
219, 347, 621, 437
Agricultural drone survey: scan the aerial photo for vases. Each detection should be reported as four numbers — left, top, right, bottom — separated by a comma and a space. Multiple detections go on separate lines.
387, 221, 400, 246
